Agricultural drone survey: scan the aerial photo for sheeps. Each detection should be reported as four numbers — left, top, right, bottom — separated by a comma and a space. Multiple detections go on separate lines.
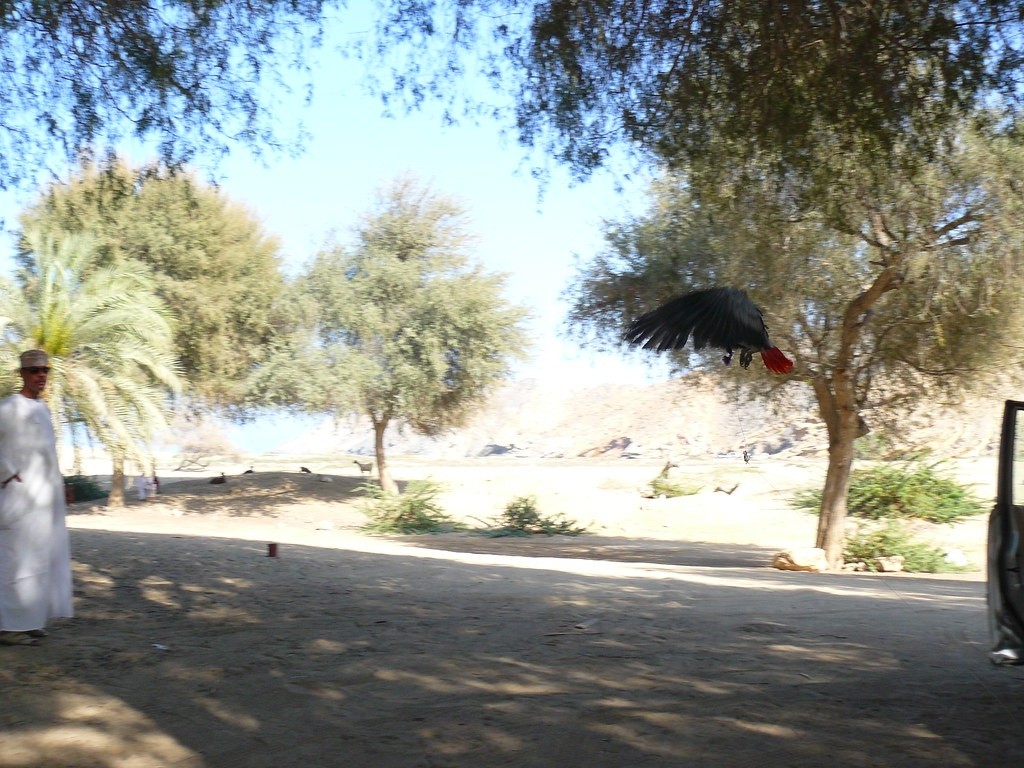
352, 460, 374, 476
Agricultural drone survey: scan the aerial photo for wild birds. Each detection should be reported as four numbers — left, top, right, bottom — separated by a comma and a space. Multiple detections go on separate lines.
713, 484, 739, 496
208, 472, 226, 484
242, 466, 255, 474
300, 466, 311, 474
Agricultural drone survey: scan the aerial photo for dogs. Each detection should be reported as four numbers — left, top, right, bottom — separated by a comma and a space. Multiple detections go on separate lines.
619, 286, 795, 377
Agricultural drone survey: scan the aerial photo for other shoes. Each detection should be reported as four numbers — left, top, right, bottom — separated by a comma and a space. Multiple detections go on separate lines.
0, 631, 39, 645
25, 628, 49, 637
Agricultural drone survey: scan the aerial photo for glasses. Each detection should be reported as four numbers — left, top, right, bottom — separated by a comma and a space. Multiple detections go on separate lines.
24, 368, 48, 374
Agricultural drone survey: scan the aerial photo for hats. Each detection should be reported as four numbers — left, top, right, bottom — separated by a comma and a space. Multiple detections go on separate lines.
21, 350, 49, 367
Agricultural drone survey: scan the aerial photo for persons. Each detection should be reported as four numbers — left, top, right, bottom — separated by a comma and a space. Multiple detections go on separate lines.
0, 349, 75, 646
